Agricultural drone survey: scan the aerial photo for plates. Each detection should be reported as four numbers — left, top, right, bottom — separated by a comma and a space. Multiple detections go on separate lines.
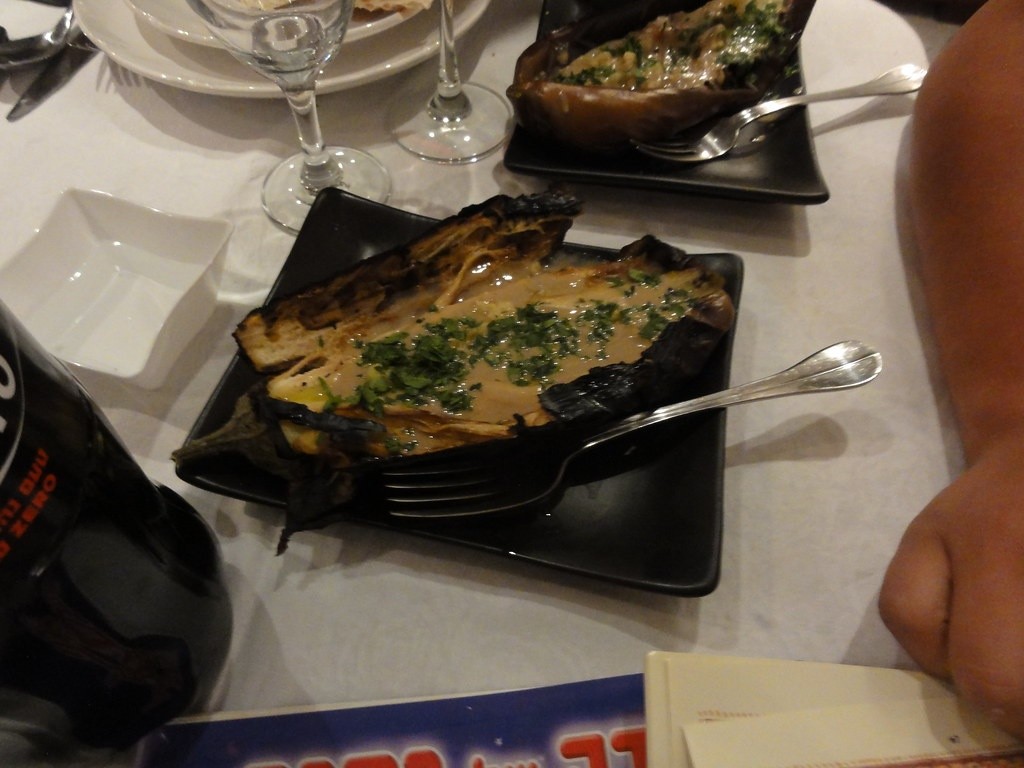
503, 0, 830, 204
124, 0, 423, 51
71, 0, 490, 99
171, 188, 743, 598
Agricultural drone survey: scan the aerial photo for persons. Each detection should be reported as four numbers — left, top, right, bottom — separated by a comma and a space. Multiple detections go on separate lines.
874, 0, 1024, 741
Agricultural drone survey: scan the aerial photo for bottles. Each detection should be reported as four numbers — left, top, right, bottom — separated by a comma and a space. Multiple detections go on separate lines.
0, 296, 230, 758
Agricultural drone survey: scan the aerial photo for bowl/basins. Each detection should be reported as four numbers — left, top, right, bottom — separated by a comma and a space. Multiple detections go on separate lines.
0, 185, 236, 390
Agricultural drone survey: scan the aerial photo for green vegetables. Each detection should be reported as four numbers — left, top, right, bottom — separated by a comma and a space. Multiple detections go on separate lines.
533, 0, 798, 98
310, 261, 695, 453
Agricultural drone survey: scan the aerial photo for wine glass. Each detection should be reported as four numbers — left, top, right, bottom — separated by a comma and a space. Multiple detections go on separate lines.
391, 0, 513, 163
189, 0, 393, 234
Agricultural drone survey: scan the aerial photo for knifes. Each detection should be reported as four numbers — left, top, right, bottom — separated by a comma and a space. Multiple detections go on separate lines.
6, 31, 101, 122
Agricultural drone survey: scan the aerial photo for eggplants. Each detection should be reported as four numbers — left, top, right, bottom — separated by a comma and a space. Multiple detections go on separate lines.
505, 0, 817, 148
166, 233, 734, 494
229, 183, 584, 370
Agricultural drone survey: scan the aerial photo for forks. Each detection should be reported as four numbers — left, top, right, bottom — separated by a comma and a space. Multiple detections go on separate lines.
628, 62, 927, 163
376, 338, 881, 518
